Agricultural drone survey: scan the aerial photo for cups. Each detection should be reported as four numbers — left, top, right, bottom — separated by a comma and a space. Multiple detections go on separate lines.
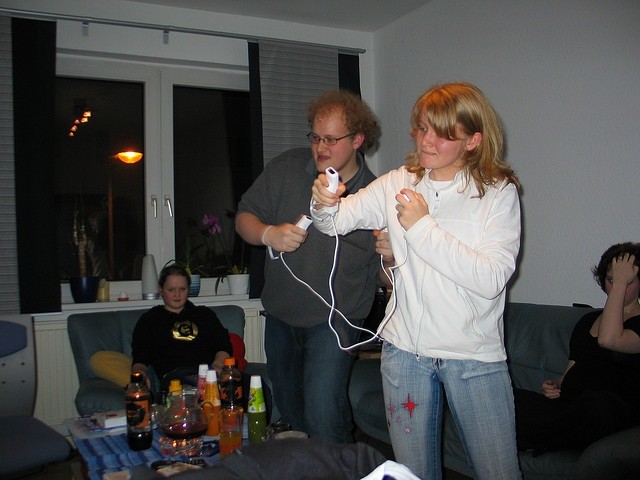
96, 280, 109, 302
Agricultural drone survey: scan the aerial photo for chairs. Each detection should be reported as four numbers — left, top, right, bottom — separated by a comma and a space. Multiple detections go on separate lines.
0, 314, 72, 480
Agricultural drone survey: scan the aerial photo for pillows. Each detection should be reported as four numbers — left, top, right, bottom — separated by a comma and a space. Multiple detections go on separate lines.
228, 332, 247, 368
89, 350, 135, 388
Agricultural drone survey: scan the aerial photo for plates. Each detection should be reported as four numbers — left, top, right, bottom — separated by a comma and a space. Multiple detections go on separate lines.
274, 429, 309, 441
117, 297, 129, 301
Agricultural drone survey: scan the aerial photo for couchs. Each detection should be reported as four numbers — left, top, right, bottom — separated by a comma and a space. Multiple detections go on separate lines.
351, 302, 639, 480
165, 441, 390, 480
66, 306, 268, 417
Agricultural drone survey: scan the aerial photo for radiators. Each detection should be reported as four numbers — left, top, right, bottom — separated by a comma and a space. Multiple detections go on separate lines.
32, 309, 263, 427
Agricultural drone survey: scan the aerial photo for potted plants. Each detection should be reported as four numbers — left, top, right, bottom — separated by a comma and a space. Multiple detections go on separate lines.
162, 232, 206, 298
65, 199, 106, 304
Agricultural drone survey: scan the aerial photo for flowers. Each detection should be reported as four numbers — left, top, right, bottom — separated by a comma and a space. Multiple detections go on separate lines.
202, 209, 244, 294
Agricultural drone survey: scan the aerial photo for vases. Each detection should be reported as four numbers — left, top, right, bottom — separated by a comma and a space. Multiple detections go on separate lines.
227, 273, 249, 298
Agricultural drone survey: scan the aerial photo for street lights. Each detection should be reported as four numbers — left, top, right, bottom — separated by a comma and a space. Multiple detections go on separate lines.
105, 139, 143, 280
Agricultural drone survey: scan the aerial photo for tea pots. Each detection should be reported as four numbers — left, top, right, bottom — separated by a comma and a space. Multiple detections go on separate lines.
151, 389, 215, 440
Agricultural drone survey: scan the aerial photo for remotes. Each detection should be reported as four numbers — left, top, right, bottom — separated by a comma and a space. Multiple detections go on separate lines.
150, 458, 207, 472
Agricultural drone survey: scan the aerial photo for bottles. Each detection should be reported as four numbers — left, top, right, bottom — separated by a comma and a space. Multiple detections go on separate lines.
246, 374, 267, 445
216, 356, 244, 409
203, 369, 222, 437
120, 290, 127, 298
139, 254, 161, 300
166, 379, 184, 414
125, 371, 153, 451
218, 399, 244, 457
70, 239, 94, 301
197, 363, 209, 404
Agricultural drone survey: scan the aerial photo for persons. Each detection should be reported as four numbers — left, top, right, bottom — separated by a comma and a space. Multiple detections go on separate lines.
307, 80, 524, 479
513, 240, 640, 460
131, 264, 235, 395
234, 85, 395, 443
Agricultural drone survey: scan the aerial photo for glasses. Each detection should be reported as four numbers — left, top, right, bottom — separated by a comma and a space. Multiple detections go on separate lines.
307, 131, 357, 145
